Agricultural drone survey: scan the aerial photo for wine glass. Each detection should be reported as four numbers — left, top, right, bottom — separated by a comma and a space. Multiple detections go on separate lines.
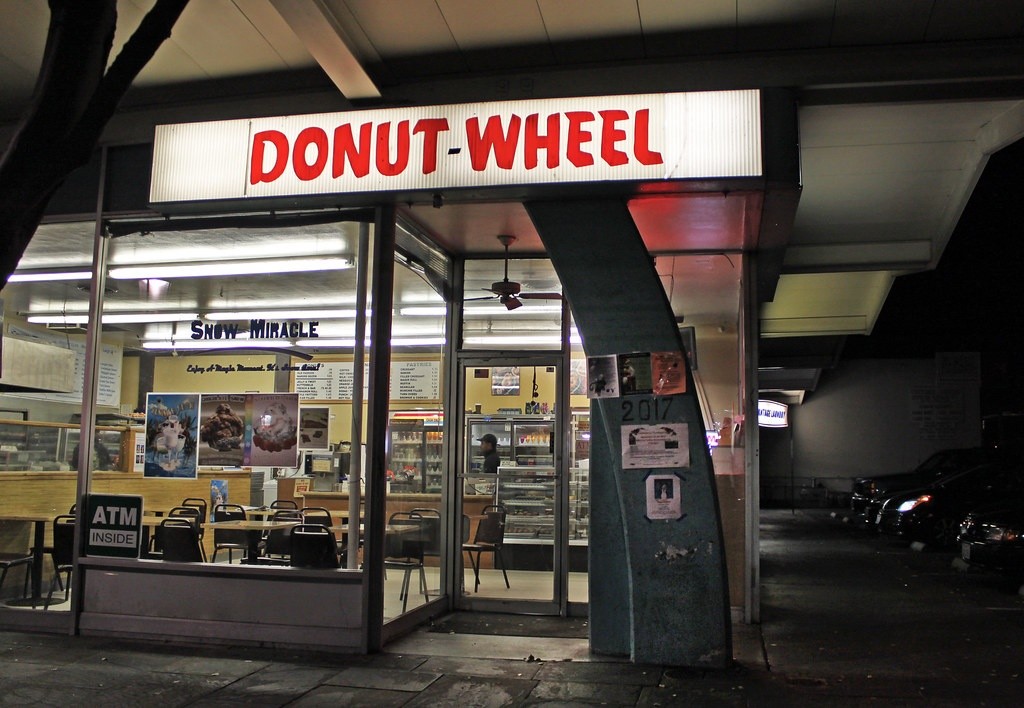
157, 414, 187, 471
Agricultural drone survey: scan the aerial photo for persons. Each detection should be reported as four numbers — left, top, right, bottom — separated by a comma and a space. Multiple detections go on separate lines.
73, 429, 112, 472
477, 434, 500, 474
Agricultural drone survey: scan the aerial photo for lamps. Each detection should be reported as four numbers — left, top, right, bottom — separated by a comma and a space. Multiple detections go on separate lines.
7, 253, 356, 280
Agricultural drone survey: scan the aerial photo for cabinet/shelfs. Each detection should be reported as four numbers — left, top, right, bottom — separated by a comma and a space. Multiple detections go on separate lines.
494, 467, 589, 573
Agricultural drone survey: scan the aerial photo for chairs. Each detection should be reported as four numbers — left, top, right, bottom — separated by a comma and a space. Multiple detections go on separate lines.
0, 498, 510, 614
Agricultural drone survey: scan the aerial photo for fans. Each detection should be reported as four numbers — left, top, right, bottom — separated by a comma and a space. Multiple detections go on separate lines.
462, 235, 564, 311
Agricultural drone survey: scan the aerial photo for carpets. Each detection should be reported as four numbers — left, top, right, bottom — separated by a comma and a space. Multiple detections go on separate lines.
427, 612, 590, 640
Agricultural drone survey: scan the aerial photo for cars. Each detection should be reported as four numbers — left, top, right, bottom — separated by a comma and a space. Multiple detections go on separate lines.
850, 412, 1023, 587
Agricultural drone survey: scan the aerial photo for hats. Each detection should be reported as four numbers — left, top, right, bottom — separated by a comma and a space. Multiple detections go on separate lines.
476, 434, 497, 443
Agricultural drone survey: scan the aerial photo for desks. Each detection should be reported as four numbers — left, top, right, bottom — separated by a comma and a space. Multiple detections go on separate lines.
412, 515, 489, 596
199, 521, 301, 565
307, 510, 365, 540
145, 506, 199, 517
0, 515, 74, 607
328, 522, 420, 535
223, 508, 298, 522
139, 516, 194, 558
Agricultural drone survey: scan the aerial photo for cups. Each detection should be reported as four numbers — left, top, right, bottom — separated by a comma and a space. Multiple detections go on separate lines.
475, 403, 482, 413
520, 434, 547, 443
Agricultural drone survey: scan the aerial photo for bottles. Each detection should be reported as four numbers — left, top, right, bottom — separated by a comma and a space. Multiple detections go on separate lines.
393, 431, 442, 485
